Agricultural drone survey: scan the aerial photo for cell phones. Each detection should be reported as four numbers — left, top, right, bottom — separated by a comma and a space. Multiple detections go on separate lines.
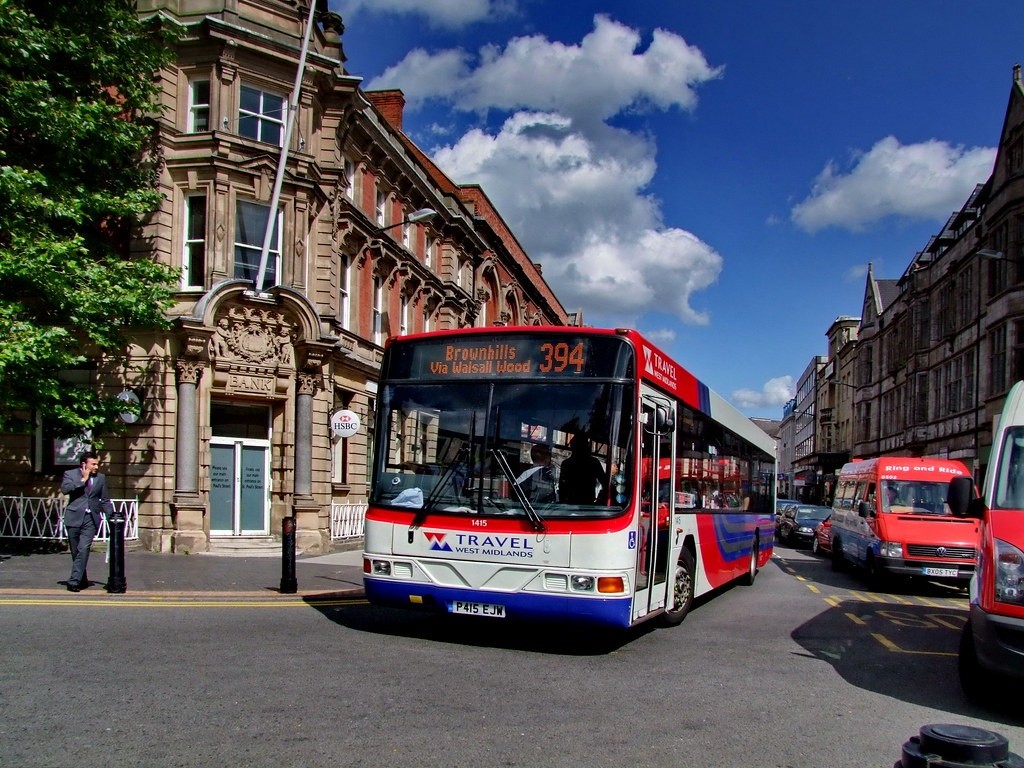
82, 462, 85, 470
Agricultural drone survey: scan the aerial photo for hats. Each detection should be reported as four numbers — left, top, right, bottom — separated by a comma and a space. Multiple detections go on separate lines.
888, 484, 899, 494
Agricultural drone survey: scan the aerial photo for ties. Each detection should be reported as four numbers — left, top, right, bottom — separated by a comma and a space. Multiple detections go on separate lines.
87, 476, 91, 491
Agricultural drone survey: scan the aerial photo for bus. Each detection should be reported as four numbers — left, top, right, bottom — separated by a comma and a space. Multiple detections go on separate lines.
360, 324, 784, 630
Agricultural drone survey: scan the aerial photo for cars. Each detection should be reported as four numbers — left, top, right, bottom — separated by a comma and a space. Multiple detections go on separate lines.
774, 498, 833, 556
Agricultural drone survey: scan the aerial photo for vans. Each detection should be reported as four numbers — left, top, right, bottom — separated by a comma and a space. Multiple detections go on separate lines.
827, 455, 982, 594
953, 379, 1024, 701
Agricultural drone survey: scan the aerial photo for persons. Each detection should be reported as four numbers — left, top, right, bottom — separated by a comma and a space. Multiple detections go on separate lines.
884, 487, 897, 507
400, 435, 492, 477
558, 435, 606, 505
62, 452, 114, 592
519, 443, 555, 504
743, 486, 750, 510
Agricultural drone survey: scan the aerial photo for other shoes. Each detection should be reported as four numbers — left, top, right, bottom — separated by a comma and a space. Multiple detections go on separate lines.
78, 579, 89, 589
67, 582, 80, 592
